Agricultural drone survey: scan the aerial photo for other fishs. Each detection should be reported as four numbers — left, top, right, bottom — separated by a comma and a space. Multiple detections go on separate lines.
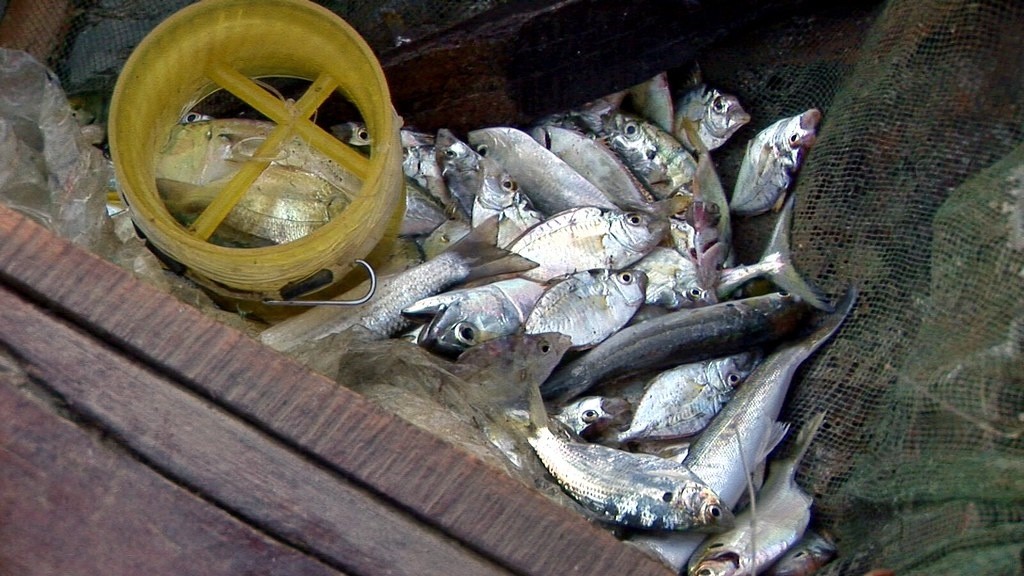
102, 62, 864, 576
